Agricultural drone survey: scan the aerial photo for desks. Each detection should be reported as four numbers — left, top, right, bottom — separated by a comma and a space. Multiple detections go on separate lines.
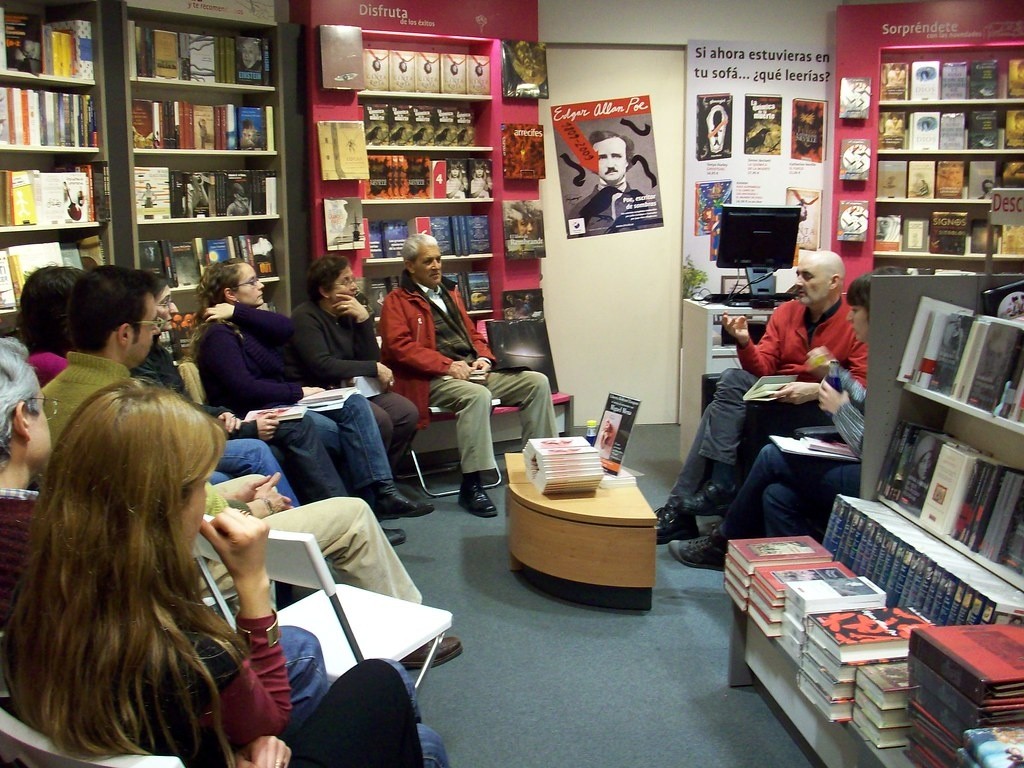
681, 298, 798, 467
504, 451, 655, 588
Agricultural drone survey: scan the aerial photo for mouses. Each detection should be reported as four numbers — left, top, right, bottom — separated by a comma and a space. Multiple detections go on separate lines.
691, 292, 704, 301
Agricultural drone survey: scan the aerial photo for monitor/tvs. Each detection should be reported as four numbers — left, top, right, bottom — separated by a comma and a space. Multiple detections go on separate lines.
716, 204, 802, 300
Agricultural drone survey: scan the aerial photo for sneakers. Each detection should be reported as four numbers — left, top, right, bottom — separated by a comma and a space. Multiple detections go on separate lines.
655, 504, 700, 543
668, 524, 724, 570
681, 482, 743, 517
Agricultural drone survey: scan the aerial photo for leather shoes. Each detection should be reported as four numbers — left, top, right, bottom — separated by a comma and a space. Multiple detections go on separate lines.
402, 636, 463, 667
458, 485, 497, 517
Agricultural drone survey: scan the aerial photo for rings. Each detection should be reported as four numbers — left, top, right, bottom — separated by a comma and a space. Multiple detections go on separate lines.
231, 415, 237, 419
275, 760, 286, 768
390, 381, 394, 386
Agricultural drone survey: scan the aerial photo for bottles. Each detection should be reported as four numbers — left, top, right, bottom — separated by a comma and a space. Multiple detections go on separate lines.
584, 420, 597, 447
823, 360, 842, 417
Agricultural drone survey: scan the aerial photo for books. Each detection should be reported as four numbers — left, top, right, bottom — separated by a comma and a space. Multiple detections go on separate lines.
722, 280, 1024, 768
440, 370, 488, 382
502, 200, 546, 259
317, 25, 492, 258
246, 406, 307, 421
0, 13, 284, 367
839, 77, 872, 120
522, 392, 642, 496
297, 387, 358, 406
837, 200, 869, 241
353, 273, 495, 321
875, 59, 1024, 256
500, 38, 550, 100
839, 139, 871, 181
501, 288, 545, 320
501, 123, 546, 180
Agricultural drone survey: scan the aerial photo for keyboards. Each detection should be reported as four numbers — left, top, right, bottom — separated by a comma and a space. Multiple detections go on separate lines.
704, 293, 797, 304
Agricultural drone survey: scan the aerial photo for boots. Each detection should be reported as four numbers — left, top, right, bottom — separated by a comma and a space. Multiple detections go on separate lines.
370, 479, 434, 519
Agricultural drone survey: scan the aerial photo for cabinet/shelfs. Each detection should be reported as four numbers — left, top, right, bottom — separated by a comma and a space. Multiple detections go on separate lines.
0, 0, 117, 339
102, 0, 292, 360
300, 26, 504, 340
729, 275, 1024, 768
866, 41, 1024, 313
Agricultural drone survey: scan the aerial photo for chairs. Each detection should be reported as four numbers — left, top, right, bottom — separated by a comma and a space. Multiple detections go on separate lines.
190, 511, 453, 697
374, 336, 501, 498
0, 704, 184, 768
477, 319, 574, 437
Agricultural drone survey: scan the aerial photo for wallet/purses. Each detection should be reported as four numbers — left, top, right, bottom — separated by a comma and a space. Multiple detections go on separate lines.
793, 426, 839, 439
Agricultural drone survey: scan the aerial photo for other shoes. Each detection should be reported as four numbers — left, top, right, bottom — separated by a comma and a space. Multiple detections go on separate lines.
384, 528, 406, 545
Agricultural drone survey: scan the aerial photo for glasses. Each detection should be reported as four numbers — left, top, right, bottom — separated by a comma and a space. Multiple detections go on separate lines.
231, 275, 259, 288
334, 277, 356, 288
114, 317, 164, 336
27, 397, 58, 420
158, 298, 174, 310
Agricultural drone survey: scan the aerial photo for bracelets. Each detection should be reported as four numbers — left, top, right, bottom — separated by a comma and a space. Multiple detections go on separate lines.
255, 497, 275, 515
235, 608, 280, 646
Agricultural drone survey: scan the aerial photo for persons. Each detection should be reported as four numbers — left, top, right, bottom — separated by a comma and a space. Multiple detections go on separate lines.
935, 316, 963, 392
378, 234, 559, 517
978, 179, 993, 200
654, 250, 868, 544
905, 436, 936, 507
668, 265, 911, 571
468, 159, 491, 198
227, 182, 251, 216
142, 183, 156, 219
978, 321, 1011, 377
884, 64, 905, 89
0, 255, 462, 768
446, 161, 468, 199
508, 211, 541, 242
235, 39, 263, 83
198, 118, 208, 148
181, 172, 208, 218
883, 113, 902, 136
240, 119, 259, 146
